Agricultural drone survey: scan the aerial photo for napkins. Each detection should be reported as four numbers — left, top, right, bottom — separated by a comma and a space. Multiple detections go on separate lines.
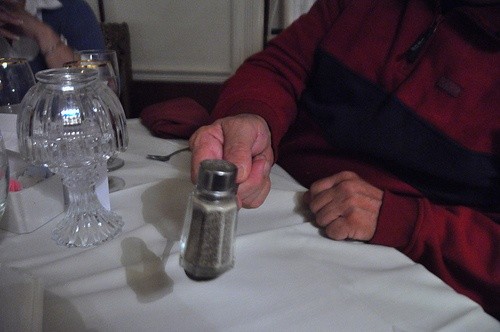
140, 96, 212, 140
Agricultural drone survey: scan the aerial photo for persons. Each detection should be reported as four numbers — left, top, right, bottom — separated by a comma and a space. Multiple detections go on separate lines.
0, 0, 107, 111
190, 0, 500, 321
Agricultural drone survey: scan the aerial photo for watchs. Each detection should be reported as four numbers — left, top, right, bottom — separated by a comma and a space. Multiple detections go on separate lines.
42, 34, 67, 55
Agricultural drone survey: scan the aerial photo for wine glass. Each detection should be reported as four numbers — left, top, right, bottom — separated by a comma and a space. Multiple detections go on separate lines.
77, 49, 125, 170
63, 61, 126, 193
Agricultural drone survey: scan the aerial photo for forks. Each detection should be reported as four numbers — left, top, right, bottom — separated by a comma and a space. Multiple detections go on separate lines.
148, 146, 190, 162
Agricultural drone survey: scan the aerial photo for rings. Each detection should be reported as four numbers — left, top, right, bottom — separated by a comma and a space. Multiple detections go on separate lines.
18, 19, 23, 26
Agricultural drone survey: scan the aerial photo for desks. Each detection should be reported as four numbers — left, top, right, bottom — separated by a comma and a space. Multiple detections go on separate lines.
0, 113, 500, 332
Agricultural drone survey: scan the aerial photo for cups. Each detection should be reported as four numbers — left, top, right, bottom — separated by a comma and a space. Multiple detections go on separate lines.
0, 58, 36, 113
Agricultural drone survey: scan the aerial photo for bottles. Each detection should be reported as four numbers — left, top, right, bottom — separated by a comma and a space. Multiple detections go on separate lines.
177, 159, 238, 278
